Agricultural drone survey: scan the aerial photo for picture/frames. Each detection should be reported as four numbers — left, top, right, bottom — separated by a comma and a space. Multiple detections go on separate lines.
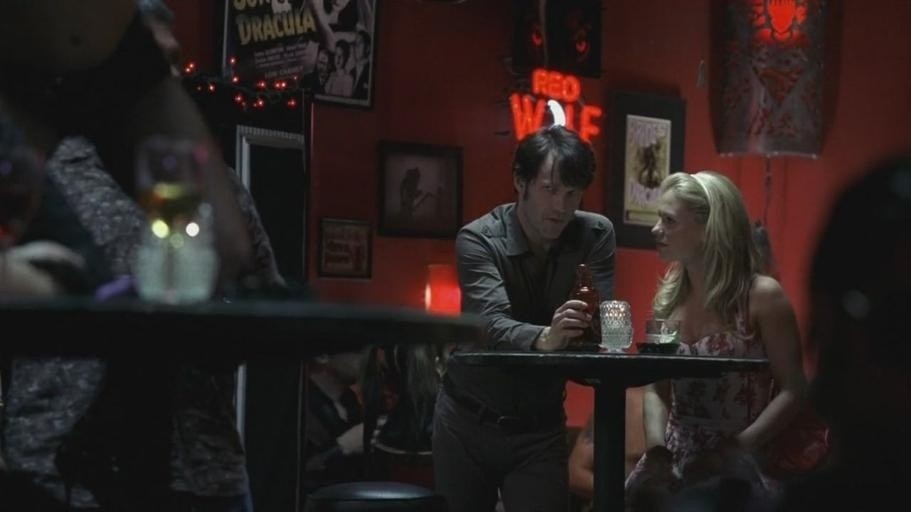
376, 139, 465, 240
316, 216, 373, 281
605, 91, 686, 250
216, 1, 381, 110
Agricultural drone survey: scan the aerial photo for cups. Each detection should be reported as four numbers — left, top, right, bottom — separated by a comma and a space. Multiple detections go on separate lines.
643, 318, 683, 343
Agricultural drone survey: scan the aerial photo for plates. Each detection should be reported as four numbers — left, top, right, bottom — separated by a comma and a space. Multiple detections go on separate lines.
636, 340, 682, 355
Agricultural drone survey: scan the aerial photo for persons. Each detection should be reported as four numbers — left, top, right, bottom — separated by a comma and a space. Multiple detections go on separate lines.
431, 124, 616, 512
624, 169, 812, 511
0, 4, 290, 511
304, 0, 373, 101
567, 385, 645, 511
306, 342, 443, 487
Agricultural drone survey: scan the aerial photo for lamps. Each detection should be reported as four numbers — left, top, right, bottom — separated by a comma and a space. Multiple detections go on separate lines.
709, 0, 843, 160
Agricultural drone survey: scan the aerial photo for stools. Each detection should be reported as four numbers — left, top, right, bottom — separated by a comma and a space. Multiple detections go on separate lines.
303, 481, 445, 512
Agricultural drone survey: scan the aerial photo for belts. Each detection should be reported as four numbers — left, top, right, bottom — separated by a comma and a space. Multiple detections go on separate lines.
443, 373, 558, 428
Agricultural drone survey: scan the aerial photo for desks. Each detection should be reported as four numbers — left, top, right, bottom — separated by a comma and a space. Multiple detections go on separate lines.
448, 349, 769, 512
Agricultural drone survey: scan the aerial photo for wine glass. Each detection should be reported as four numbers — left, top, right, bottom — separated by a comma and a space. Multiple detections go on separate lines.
128, 134, 220, 305
597, 297, 634, 353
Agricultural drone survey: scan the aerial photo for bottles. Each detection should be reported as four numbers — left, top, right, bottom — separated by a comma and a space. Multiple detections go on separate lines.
568, 263, 602, 354
